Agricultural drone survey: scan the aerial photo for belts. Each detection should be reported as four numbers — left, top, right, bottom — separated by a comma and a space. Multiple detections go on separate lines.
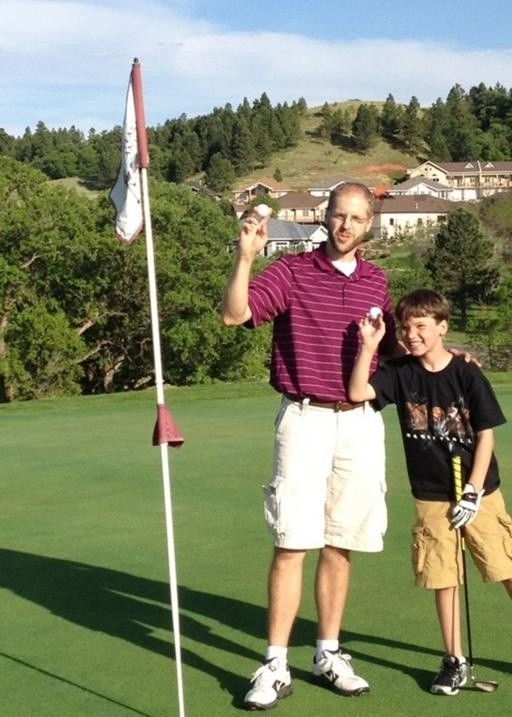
281, 391, 377, 413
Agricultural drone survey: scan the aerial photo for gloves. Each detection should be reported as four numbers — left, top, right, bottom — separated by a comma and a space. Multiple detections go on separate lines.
447, 483, 486, 531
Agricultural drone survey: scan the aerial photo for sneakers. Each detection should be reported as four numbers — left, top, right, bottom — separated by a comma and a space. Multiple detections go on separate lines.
241, 656, 295, 713
429, 653, 470, 697
309, 646, 372, 697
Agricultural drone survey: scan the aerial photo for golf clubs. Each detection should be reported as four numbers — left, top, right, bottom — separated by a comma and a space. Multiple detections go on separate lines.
452, 455, 499, 693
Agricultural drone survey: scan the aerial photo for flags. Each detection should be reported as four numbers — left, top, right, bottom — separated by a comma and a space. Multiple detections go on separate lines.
108, 63, 149, 246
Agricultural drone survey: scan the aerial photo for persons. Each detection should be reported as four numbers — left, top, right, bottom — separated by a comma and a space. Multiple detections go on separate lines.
218, 179, 484, 712
345, 286, 512, 698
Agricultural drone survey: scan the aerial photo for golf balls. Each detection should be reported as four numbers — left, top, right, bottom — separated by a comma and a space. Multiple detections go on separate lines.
256, 203, 270, 217
369, 307, 382, 319
